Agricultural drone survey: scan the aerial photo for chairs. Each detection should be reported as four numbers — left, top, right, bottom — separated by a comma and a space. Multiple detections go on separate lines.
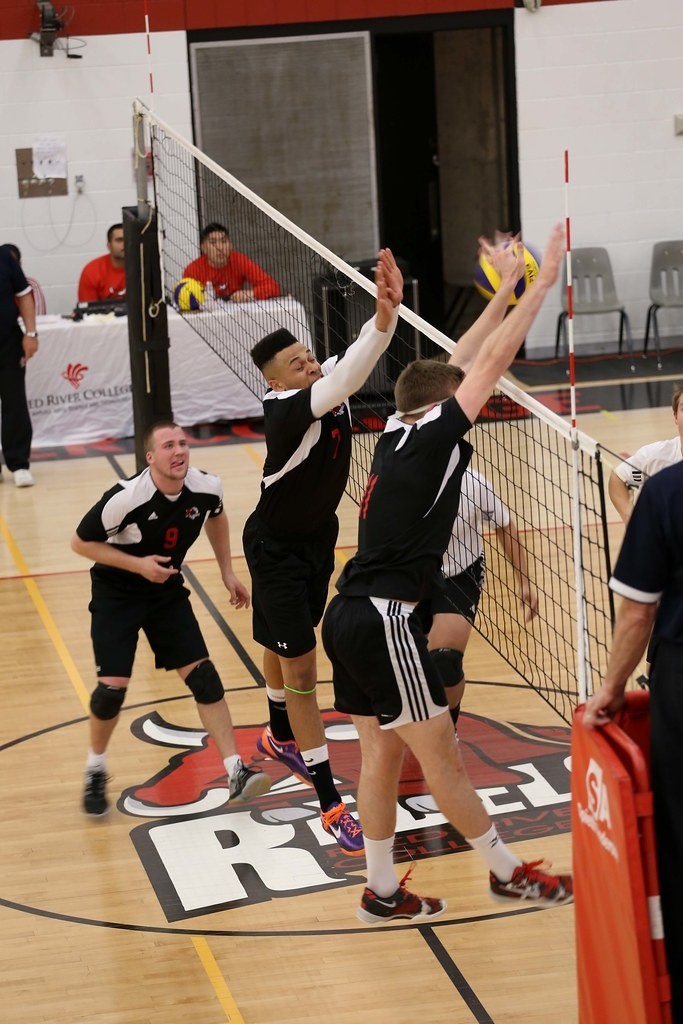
554, 248, 636, 375
642, 240, 683, 372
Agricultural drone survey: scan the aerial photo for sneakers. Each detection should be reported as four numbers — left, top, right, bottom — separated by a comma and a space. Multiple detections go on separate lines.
13, 468, 33, 486
355, 869, 447, 923
82, 770, 109, 816
257, 723, 314, 786
320, 802, 366, 856
228, 757, 272, 804
489, 859, 575, 908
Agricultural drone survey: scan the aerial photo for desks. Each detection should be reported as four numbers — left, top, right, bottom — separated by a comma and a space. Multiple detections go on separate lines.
18, 296, 315, 449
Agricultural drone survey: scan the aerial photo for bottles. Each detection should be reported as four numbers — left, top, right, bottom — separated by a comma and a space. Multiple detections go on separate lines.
204, 281, 214, 309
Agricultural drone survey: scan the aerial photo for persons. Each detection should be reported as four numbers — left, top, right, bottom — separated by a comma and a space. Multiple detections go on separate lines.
420, 465, 540, 742
608, 386, 683, 525
322, 222, 575, 926
71, 419, 272, 818
241, 246, 402, 857
184, 222, 281, 303
582, 462, 683, 1024
0, 244, 46, 315
76, 224, 127, 302
0, 248, 39, 488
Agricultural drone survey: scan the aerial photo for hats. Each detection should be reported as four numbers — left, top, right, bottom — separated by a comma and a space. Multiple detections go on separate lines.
251, 328, 296, 370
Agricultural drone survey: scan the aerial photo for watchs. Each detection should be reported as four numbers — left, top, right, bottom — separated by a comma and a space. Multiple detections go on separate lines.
26, 331, 38, 338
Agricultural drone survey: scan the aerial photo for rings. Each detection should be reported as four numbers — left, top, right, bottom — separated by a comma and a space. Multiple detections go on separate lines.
584, 713, 593, 720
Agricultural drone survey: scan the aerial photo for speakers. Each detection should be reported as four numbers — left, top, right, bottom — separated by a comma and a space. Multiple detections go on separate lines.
315, 277, 422, 398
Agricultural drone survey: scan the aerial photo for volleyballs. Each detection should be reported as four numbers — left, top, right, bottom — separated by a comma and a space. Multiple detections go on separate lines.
172, 277, 206, 311
469, 239, 540, 305
